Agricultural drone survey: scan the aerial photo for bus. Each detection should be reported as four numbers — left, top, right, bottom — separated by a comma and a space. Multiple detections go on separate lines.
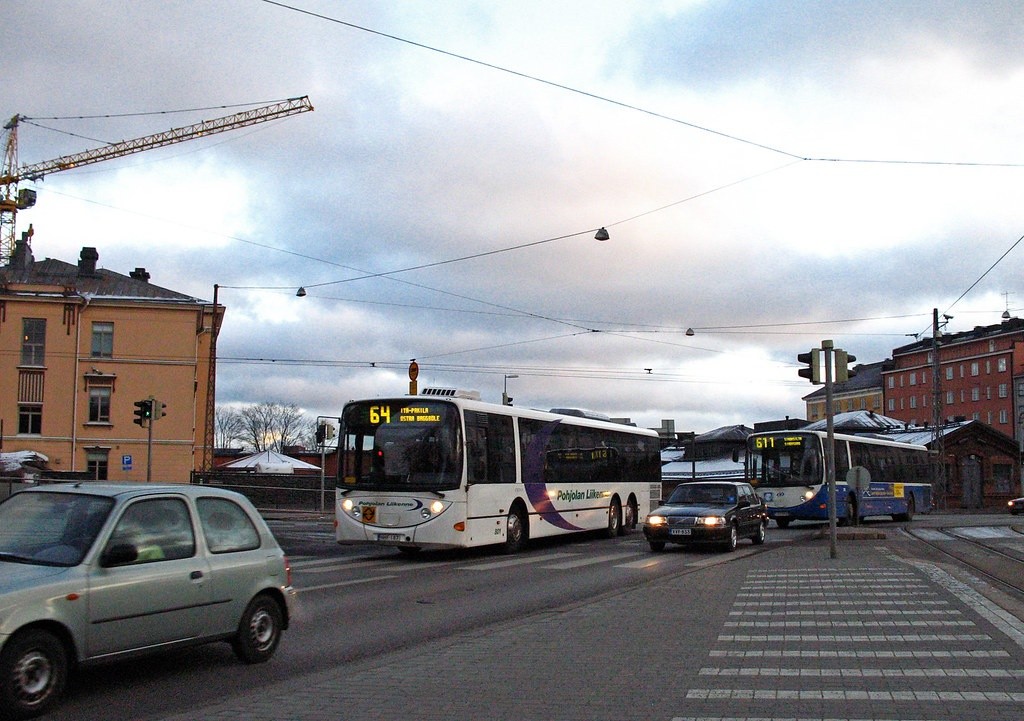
314, 386, 663, 557
732, 435, 935, 527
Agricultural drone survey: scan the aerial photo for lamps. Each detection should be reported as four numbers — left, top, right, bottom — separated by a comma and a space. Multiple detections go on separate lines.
1002, 311, 1011, 318
594, 227, 610, 241
686, 328, 694, 336
295, 287, 306, 296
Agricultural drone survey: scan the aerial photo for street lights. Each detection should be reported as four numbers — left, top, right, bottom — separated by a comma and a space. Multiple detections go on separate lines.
504, 374, 519, 394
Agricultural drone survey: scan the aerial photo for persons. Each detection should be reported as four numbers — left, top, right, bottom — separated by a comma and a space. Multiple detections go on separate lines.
111, 521, 162, 561
719, 488, 737, 504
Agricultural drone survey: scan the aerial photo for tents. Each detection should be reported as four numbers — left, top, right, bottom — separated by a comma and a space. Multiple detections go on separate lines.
228, 450, 323, 510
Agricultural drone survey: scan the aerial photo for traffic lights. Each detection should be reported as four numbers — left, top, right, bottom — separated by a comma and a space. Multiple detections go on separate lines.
834, 348, 857, 382
798, 348, 820, 382
155, 399, 167, 419
133, 400, 146, 427
503, 392, 514, 406
142, 400, 153, 418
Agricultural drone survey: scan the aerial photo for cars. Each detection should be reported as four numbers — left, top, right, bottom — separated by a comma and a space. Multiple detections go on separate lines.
1008, 498, 1024, 515
643, 480, 769, 553
0, 478, 298, 721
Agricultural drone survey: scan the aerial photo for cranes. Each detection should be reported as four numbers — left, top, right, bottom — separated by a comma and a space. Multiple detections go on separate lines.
0, 95, 313, 285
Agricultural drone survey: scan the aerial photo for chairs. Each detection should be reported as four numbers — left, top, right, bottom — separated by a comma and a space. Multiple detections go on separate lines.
688, 490, 703, 502
209, 513, 238, 549
72, 512, 107, 547
146, 509, 181, 556
